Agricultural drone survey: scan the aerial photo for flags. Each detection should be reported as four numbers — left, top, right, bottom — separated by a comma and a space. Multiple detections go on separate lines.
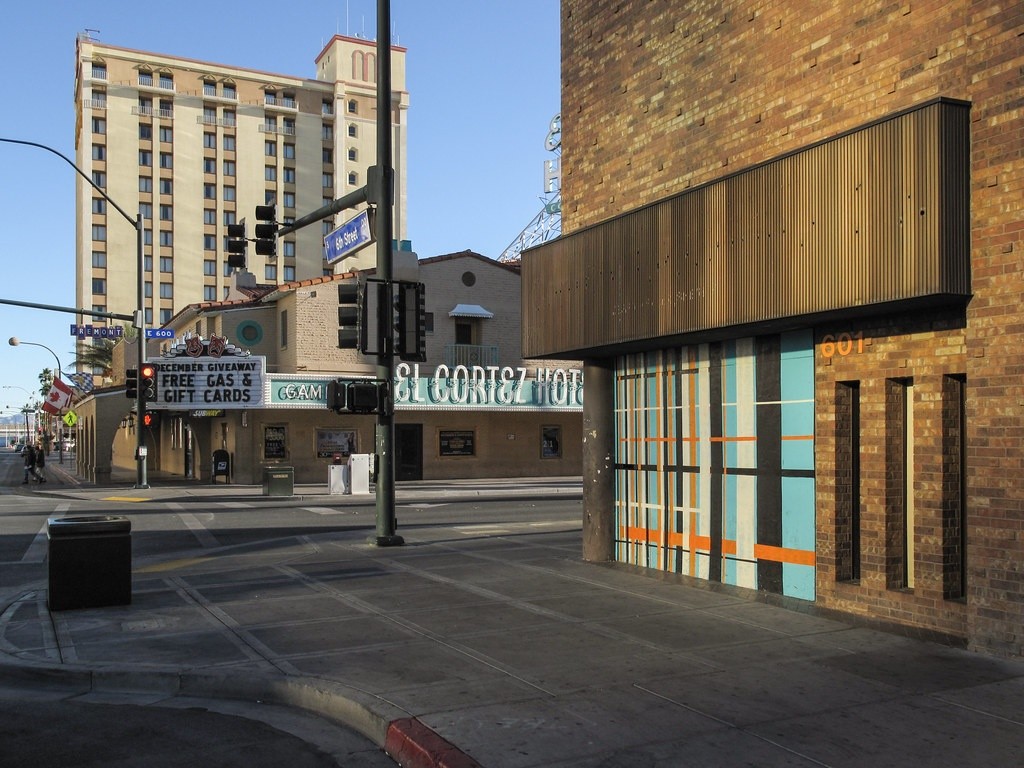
41, 377, 72, 415
66, 372, 94, 391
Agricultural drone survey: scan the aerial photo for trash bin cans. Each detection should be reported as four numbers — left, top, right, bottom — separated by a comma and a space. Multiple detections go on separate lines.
43, 516, 133, 612
262, 466, 294, 497
211, 449, 231, 484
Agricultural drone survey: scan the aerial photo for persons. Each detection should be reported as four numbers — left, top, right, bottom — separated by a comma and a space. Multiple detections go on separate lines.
32, 446, 47, 482
22, 445, 43, 484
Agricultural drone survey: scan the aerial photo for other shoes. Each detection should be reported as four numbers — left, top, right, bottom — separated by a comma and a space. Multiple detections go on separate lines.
22, 481, 28, 485
38, 477, 43, 484
32, 479, 38, 482
42, 479, 47, 483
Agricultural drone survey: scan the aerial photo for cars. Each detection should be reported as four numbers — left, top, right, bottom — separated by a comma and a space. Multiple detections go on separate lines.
14, 443, 36, 458
53, 438, 75, 452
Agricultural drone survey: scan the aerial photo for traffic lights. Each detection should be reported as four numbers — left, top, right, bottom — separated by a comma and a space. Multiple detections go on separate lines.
145, 412, 158, 428
254, 198, 277, 260
326, 379, 345, 412
38, 428, 42, 435
125, 369, 137, 399
228, 217, 247, 273
142, 363, 158, 401
337, 281, 385, 353
393, 279, 417, 358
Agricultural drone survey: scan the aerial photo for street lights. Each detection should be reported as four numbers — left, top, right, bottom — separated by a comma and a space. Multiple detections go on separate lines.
0, 405, 26, 445
8, 336, 63, 464
3, 385, 42, 449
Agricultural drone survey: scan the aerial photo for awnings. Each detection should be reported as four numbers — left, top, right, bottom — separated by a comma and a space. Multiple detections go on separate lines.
449, 304, 494, 318
